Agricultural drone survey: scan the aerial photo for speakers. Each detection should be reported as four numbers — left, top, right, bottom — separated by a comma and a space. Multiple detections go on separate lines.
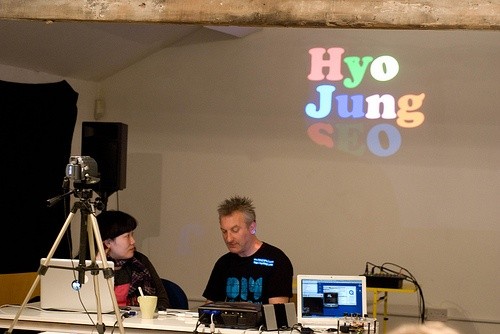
80, 121, 128, 191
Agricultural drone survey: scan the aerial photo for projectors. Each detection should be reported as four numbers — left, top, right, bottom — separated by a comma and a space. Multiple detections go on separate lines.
198, 301, 262, 329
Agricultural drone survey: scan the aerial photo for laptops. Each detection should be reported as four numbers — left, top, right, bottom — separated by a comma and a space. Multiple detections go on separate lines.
294, 275, 369, 332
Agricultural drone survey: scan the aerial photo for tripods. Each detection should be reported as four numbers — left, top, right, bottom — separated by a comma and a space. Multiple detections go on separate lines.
4, 202, 123, 333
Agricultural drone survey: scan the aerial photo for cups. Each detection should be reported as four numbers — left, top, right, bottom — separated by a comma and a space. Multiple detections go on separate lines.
138, 296, 158, 320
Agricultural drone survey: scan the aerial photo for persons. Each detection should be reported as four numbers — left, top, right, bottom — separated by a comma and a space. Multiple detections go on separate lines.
86, 209, 169, 312
202, 196, 293, 306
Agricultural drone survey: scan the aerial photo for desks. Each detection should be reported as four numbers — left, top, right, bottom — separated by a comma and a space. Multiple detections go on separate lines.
0, 302, 380, 334
367, 283, 416, 320
